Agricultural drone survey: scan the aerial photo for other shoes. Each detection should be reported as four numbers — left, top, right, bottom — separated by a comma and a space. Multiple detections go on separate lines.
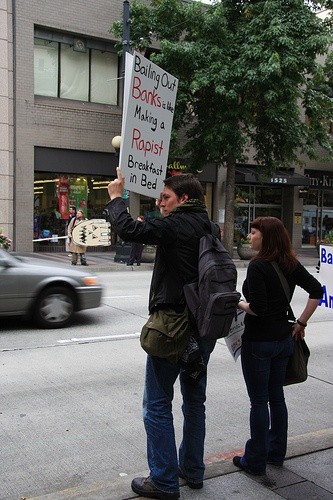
72, 261, 77, 265
81, 261, 87, 265
131, 476, 180, 500
178, 464, 203, 489
127, 263, 133, 266
137, 263, 140, 265
233, 456, 241, 467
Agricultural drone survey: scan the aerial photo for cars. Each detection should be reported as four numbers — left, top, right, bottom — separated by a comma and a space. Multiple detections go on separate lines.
0, 249, 104, 328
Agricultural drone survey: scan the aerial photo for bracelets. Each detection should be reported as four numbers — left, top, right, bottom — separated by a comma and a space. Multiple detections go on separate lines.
296, 319, 307, 327
236, 299, 244, 309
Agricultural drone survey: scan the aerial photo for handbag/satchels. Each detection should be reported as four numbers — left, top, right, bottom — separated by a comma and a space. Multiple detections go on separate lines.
66, 238, 71, 251
139, 309, 190, 362
281, 321, 311, 387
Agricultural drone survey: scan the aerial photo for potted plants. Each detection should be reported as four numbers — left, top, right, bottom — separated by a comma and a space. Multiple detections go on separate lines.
237, 233, 260, 260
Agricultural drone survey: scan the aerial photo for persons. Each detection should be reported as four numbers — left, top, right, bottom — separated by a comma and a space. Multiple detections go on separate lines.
315, 238, 327, 273
127, 216, 145, 265
66, 206, 89, 266
108, 167, 220, 500
318, 215, 331, 234
236, 217, 324, 476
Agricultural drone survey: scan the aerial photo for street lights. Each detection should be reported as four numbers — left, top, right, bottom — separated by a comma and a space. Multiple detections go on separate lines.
110, 136, 121, 250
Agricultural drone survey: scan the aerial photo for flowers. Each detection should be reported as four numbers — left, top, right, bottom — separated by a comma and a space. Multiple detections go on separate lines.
0, 226, 12, 250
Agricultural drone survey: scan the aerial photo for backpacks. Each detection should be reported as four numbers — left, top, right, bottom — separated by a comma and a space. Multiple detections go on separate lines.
182, 213, 241, 340
